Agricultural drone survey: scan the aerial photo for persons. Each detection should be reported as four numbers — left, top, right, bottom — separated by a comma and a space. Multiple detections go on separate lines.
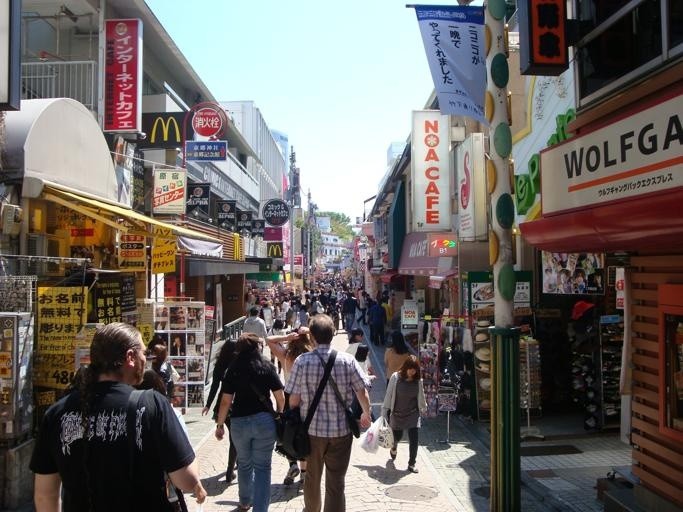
215, 333, 285, 511
265, 327, 314, 486
150, 344, 181, 407
284, 313, 372, 512
132, 368, 190, 512
383, 356, 427, 473
243, 277, 410, 390
201, 340, 238, 483
544, 253, 603, 294
28, 321, 198, 512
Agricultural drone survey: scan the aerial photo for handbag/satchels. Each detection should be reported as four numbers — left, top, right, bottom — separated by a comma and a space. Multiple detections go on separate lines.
379, 426, 391, 449
316, 307, 324, 314
281, 407, 309, 460
346, 407, 361, 438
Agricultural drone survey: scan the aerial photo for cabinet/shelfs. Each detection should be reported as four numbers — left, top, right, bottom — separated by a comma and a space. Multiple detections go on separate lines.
599, 320, 625, 429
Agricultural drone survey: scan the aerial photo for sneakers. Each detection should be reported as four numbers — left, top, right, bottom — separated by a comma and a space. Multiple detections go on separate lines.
225, 474, 235, 483
390, 447, 397, 460
284, 464, 306, 491
407, 465, 417, 471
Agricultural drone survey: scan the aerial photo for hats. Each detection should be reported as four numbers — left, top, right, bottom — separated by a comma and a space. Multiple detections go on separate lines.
474, 333, 488, 344
474, 319, 491, 329
571, 301, 593, 319
478, 399, 490, 410
474, 349, 491, 372
478, 376, 490, 391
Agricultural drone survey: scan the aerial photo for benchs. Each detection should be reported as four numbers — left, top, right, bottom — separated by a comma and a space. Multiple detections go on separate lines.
604, 486, 683, 512
